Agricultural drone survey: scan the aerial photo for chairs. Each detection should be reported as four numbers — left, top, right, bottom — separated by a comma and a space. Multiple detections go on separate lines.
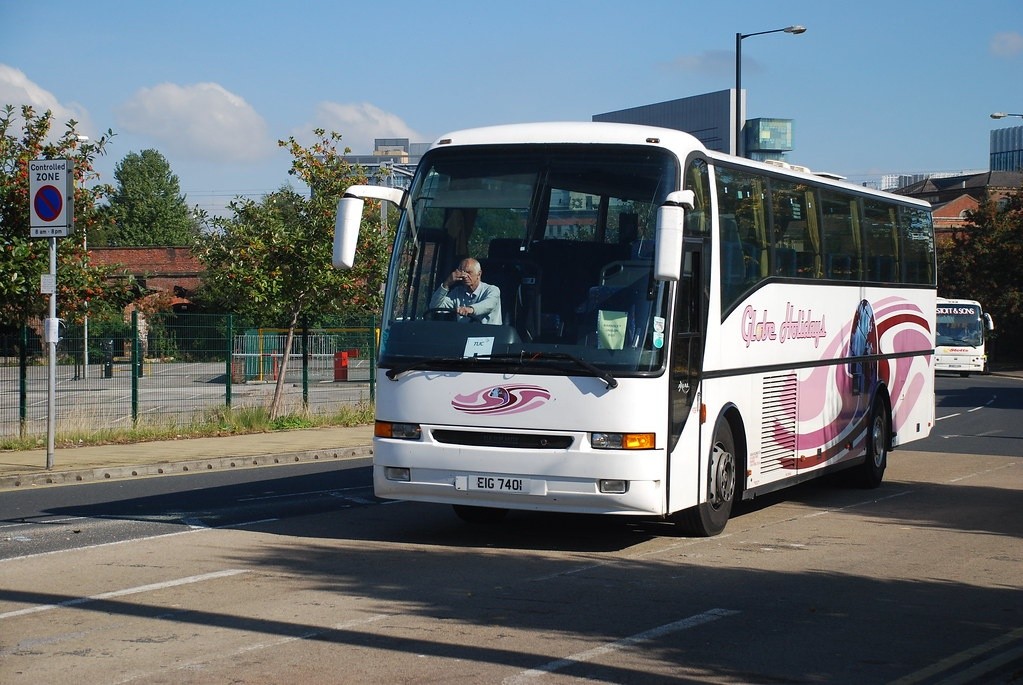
578, 237, 903, 351
482, 260, 523, 331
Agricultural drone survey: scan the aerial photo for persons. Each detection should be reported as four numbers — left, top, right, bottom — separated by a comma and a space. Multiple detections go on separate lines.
430, 259, 503, 326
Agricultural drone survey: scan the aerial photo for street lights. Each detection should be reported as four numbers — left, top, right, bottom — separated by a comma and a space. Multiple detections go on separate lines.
736, 25, 808, 157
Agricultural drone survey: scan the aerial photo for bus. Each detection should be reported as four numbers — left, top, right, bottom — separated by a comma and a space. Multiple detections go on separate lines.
331, 121, 939, 537
933, 296, 995, 377
